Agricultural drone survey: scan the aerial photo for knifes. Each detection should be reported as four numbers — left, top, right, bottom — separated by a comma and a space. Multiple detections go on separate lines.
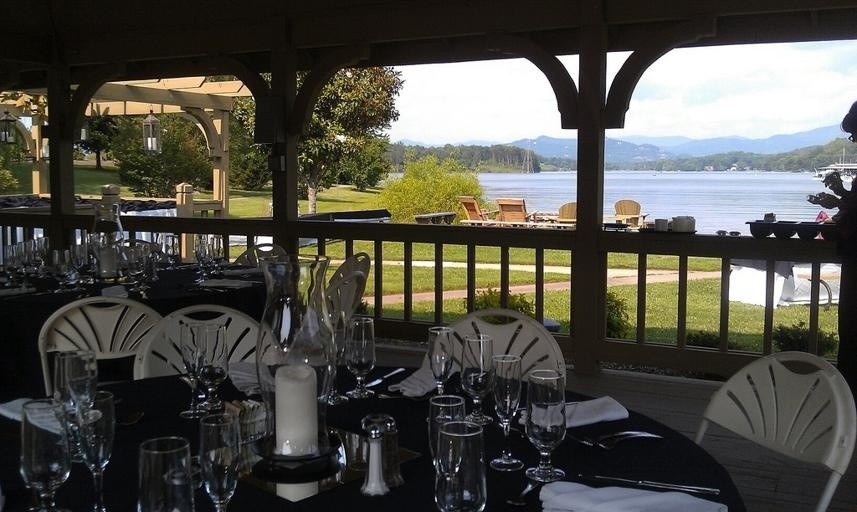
366, 368, 407, 388
577, 469, 720, 499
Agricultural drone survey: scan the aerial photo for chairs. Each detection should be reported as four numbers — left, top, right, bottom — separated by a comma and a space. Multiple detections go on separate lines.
234, 243, 289, 267
134, 304, 283, 381
695, 351, 856, 512
39, 297, 163, 397
423, 309, 567, 389
330, 252, 371, 287
325, 270, 367, 329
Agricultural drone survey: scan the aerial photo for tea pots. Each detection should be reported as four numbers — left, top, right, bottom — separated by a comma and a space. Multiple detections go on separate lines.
671, 216, 695, 234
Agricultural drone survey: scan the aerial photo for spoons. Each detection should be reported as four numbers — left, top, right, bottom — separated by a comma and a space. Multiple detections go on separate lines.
505, 480, 537, 505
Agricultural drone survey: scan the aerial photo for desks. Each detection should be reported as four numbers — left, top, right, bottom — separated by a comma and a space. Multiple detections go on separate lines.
1, 265, 280, 390
2, 365, 741, 512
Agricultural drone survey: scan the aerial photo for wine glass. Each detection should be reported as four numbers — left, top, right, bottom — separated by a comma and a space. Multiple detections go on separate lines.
1, 233, 227, 287
74, 391, 116, 509
198, 416, 242, 512
426, 326, 453, 403
320, 315, 346, 404
461, 336, 494, 426
54, 349, 99, 468
525, 370, 566, 482
346, 318, 376, 401
181, 320, 229, 424
22, 400, 70, 507
488, 356, 522, 471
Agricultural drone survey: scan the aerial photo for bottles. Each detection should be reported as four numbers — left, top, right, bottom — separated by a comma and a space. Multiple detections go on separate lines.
361, 425, 388, 495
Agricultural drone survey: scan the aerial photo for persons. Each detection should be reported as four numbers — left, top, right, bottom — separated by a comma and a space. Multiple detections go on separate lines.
804, 99, 856, 393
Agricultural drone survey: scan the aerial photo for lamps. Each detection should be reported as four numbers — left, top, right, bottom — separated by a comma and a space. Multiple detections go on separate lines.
142, 103, 161, 156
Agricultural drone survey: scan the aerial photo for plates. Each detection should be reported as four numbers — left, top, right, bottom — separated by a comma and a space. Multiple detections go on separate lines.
253, 463, 339, 482
251, 431, 341, 462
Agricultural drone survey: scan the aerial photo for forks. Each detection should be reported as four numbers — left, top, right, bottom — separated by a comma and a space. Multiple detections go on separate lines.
572, 428, 667, 451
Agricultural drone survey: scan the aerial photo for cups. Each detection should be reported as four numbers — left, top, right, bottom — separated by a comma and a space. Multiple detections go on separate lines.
436, 422, 486, 508
430, 396, 468, 494
654, 218, 668, 231
140, 437, 191, 512
